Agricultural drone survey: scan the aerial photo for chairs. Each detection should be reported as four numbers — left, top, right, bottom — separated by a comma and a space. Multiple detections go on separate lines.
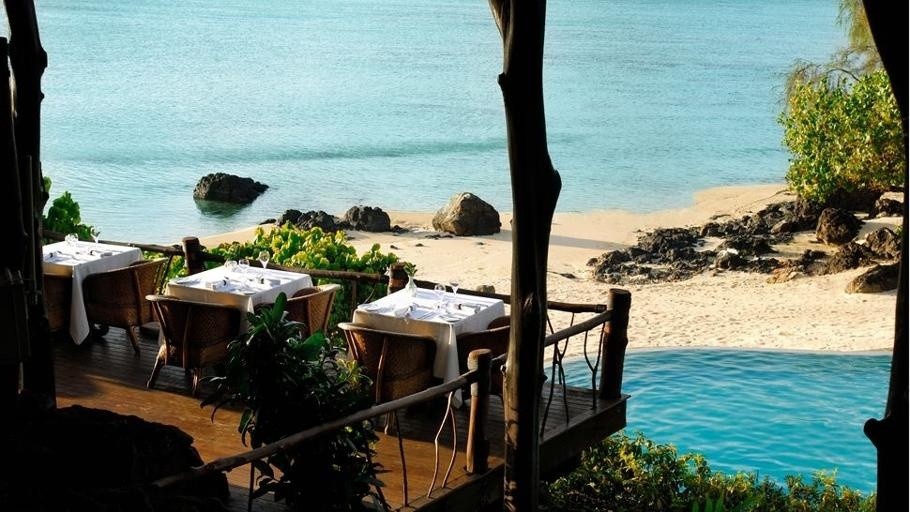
335, 320, 443, 441
458, 315, 511, 410
250, 282, 340, 381
149, 292, 237, 399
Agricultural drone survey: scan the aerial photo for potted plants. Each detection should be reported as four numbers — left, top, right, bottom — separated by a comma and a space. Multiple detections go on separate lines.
403, 263, 420, 297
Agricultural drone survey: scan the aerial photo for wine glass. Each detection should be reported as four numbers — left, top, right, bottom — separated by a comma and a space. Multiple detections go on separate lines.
224, 250, 271, 283
435, 280, 460, 312
65, 224, 101, 254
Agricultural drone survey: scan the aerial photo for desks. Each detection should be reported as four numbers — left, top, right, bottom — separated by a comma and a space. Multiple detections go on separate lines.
166, 263, 313, 371
354, 286, 506, 415
